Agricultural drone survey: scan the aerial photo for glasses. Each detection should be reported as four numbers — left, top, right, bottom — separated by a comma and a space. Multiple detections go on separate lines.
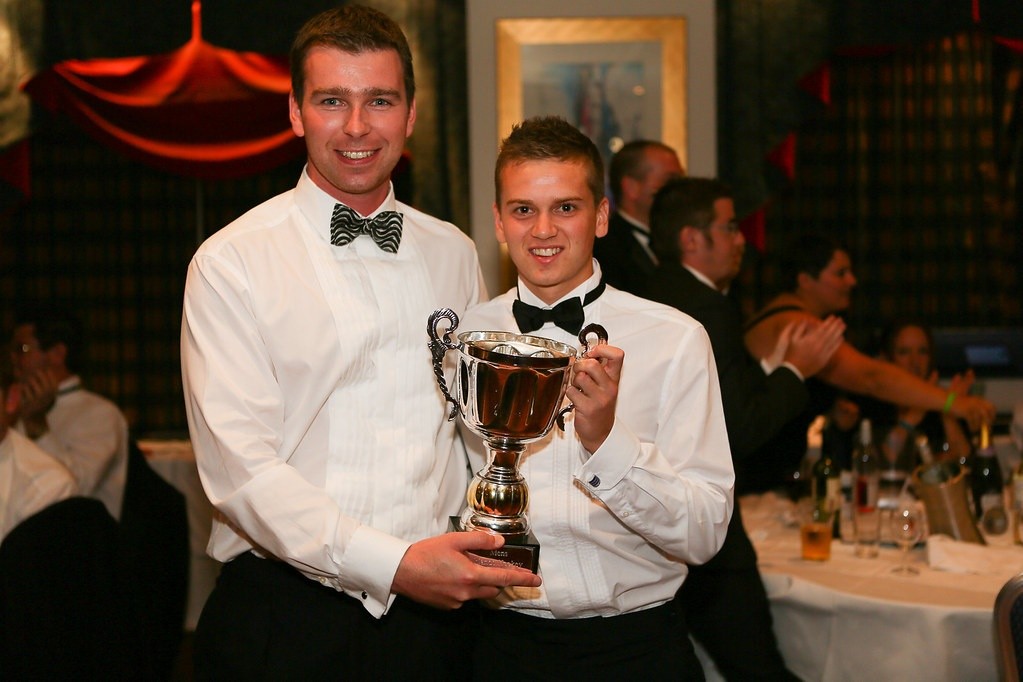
707, 221, 739, 236
12, 342, 36, 355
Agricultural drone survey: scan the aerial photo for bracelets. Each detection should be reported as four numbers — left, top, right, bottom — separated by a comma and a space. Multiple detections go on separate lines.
896, 416, 918, 432
943, 392, 959, 414
883, 436, 905, 451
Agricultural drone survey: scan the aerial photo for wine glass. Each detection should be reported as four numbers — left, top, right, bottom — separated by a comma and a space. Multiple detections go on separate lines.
889, 508, 923, 577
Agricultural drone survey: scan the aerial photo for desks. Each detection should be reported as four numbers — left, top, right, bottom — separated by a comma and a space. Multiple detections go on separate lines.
137, 439, 215, 631
694, 496, 1023, 682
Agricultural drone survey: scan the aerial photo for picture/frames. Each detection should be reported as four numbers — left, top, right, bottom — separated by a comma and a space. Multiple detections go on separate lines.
496, 14, 690, 295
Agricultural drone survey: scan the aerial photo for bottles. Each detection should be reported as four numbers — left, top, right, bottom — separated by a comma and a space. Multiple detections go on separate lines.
851, 418, 882, 519
914, 436, 950, 485
811, 429, 842, 538
970, 420, 1003, 517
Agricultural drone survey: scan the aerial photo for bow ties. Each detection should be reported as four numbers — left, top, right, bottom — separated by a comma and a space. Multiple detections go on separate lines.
329, 204, 403, 254
649, 241, 657, 254
509, 298, 586, 336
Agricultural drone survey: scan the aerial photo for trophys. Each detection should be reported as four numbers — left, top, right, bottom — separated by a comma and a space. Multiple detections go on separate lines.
425, 307, 608, 589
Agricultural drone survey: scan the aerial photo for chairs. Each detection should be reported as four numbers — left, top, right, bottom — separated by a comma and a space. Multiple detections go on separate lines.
0, 494, 124, 682
119, 437, 191, 682
993, 572, 1023, 682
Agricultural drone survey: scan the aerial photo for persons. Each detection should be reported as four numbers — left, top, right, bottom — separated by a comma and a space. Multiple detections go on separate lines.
0, 316, 129, 555
451, 113, 734, 682
592, 137, 997, 682
180, 3, 543, 682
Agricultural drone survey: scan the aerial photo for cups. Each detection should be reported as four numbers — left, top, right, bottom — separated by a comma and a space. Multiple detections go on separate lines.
853, 508, 880, 558
798, 500, 836, 560
